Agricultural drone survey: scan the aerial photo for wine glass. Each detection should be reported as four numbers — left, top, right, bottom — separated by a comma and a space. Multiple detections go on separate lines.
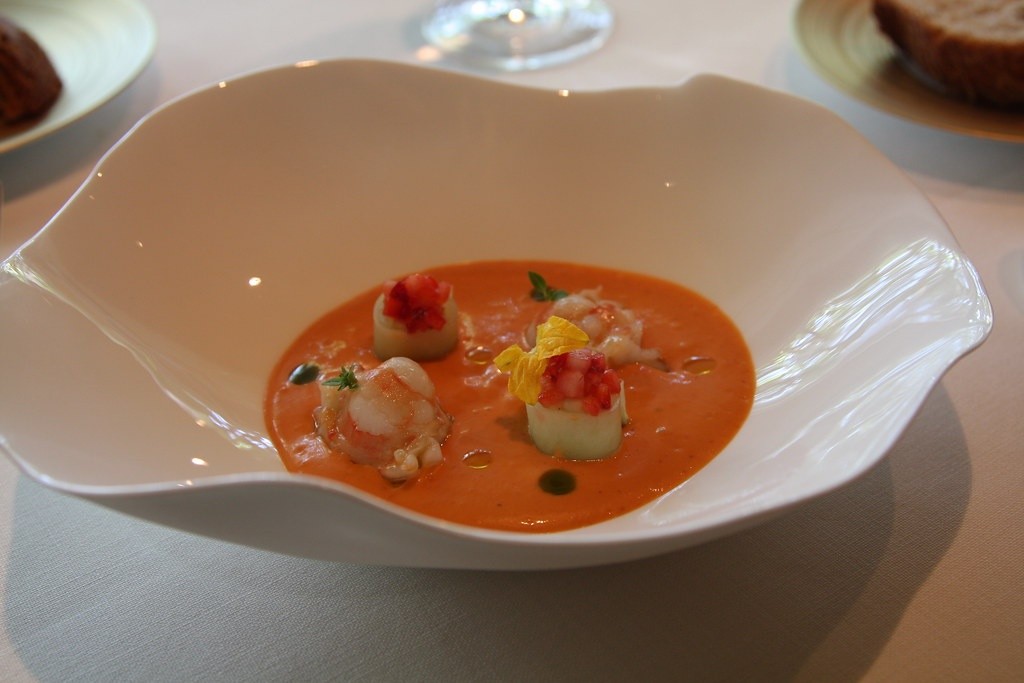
419, 2, 614, 67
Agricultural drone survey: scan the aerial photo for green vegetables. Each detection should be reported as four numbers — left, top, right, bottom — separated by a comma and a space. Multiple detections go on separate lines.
527, 269, 569, 300
320, 363, 359, 392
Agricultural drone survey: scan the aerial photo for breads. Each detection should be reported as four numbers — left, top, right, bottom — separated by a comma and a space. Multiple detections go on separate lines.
0, 16, 64, 126
870, 0, 1024, 117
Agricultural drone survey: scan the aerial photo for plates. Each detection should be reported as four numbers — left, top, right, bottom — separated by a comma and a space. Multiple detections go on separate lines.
792, 0, 1023, 146
0, 0, 159, 159
1, 61, 992, 569
